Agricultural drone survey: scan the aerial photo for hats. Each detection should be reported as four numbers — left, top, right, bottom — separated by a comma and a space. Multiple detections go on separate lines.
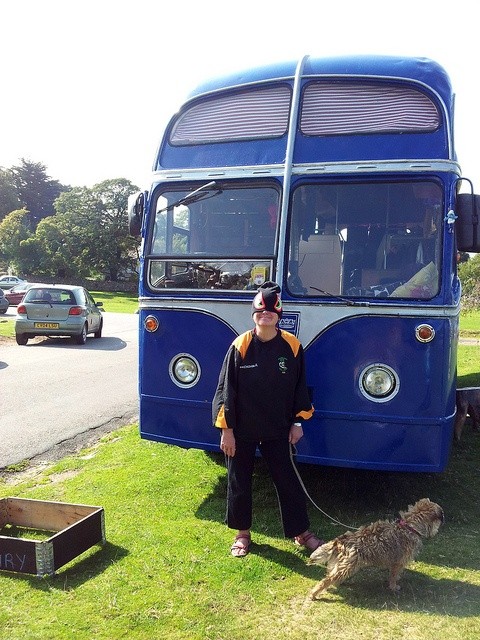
251, 281, 283, 318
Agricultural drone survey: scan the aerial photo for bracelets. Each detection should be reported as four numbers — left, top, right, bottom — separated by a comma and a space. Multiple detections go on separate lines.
294, 422, 303, 427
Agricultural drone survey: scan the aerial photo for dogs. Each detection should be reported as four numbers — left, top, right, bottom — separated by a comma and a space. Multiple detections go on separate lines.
309, 497, 445, 601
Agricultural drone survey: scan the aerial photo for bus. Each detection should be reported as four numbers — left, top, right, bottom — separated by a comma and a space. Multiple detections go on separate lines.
128, 55, 480, 472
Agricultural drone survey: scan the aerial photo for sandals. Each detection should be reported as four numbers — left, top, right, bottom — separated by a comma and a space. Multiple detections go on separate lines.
231, 534, 251, 557
294, 532, 324, 550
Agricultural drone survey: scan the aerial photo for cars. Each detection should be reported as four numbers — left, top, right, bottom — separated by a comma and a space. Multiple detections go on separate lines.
4, 282, 44, 307
0, 288, 10, 314
15, 283, 103, 345
0, 275, 27, 288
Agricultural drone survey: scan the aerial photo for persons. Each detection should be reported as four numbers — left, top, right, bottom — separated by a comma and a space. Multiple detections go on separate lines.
213, 281, 326, 557
